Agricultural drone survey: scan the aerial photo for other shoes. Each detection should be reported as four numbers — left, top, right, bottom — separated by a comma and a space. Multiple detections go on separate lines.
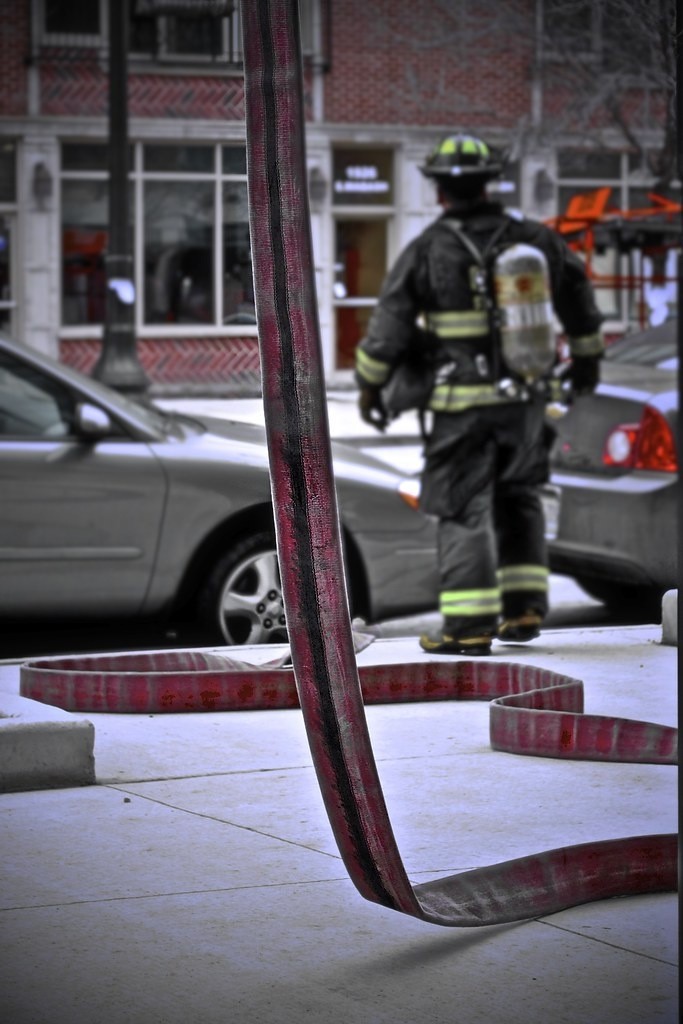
497, 609, 541, 642
421, 616, 496, 656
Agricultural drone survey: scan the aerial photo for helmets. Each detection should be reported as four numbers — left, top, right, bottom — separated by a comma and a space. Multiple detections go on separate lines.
420, 135, 501, 178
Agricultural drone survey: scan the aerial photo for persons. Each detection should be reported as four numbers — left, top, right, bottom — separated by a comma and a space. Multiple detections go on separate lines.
356, 133, 604, 654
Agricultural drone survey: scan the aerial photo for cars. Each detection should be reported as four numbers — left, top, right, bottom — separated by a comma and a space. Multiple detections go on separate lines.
0, 332, 443, 652
534, 311, 683, 612
